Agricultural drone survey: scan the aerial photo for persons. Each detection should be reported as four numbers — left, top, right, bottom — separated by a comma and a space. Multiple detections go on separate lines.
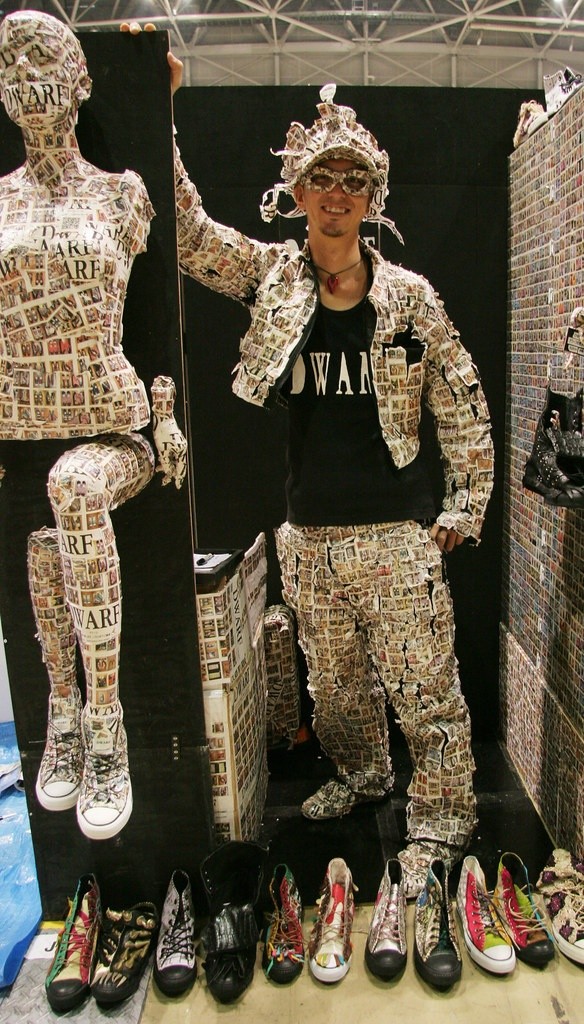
121, 22, 495, 891
0, 10, 185, 841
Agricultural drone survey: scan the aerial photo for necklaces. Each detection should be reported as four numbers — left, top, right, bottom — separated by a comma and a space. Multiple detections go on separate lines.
314, 255, 363, 295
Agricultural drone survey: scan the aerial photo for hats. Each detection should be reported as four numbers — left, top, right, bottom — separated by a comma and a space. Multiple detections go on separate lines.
274, 82, 390, 216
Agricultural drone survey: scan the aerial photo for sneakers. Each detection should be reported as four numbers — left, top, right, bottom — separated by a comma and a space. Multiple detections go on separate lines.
78, 704, 134, 840
36, 684, 82, 811
44, 840, 584, 1014
543, 67, 584, 120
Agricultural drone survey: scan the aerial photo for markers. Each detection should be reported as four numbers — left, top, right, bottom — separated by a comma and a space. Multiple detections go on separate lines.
197, 553, 215, 566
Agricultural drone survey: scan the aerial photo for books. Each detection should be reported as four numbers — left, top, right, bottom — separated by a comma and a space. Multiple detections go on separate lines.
196, 554, 231, 574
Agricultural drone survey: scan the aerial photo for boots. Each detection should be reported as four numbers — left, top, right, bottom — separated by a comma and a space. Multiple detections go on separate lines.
520, 387, 584, 509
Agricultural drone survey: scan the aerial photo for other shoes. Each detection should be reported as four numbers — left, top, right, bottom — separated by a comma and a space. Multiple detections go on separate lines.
300, 774, 386, 820
512, 99, 547, 146
397, 838, 467, 899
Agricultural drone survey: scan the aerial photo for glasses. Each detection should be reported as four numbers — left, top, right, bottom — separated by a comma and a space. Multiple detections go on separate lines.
302, 169, 373, 196
